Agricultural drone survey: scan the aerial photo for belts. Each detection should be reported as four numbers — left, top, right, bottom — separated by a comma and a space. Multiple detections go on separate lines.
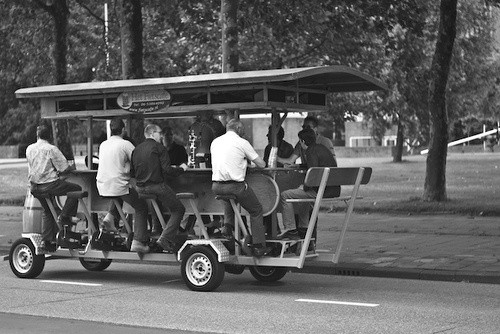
212, 180, 244, 184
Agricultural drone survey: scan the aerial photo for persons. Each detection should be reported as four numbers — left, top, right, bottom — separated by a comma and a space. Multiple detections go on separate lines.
25, 125, 83, 249
264, 124, 293, 167
96, 119, 150, 253
210, 119, 271, 257
278, 117, 337, 166
279, 129, 341, 237
132, 123, 185, 252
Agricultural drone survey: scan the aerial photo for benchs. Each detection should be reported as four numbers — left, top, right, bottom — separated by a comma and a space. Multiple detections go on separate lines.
285, 167, 374, 209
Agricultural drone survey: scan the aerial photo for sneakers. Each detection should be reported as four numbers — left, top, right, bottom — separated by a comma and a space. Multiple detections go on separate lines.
103, 213, 117, 232
277, 230, 301, 240
129, 240, 151, 252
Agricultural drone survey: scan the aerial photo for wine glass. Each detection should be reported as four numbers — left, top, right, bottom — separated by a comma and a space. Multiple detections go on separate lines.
68, 160, 74, 167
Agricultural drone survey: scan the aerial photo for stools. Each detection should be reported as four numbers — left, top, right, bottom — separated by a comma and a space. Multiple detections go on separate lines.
66, 191, 97, 233
215, 194, 251, 242
175, 192, 210, 240
37, 193, 66, 237
138, 193, 167, 231
101, 196, 132, 236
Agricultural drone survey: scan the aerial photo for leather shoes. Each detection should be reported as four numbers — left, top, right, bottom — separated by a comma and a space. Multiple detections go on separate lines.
38, 240, 55, 252
155, 236, 176, 251
251, 245, 269, 257
220, 224, 233, 236
57, 215, 77, 226
